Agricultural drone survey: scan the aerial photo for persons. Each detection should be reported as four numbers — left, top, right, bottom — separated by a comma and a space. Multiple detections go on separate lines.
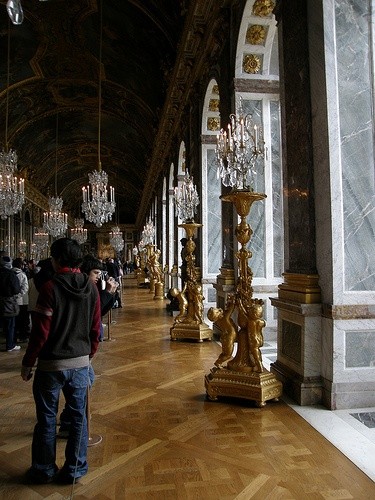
208, 297, 266, 372
170, 282, 204, 325
0, 238, 125, 486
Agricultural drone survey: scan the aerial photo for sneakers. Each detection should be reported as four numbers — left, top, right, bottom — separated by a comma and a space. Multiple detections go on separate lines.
7, 345, 21, 352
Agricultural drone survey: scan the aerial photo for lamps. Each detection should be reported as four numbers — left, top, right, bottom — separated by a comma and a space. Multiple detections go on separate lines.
0, 19, 25, 220
43, 74, 68, 237
111, 205, 125, 252
171, 167, 200, 225
215, 114, 265, 194
141, 221, 157, 244
19, 228, 49, 260
82, 0, 117, 228
71, 198, 88, 244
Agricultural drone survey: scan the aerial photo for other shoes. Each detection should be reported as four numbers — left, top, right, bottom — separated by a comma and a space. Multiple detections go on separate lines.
100, 336, 109, 342
22, 466, 59, 485
58, 420, 72, 439
54, 471, 78, 485
17, 337, 30, 343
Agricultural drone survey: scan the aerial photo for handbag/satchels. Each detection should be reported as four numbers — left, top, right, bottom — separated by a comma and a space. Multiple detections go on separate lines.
0, 295, 20, 317
101, 273, 106, 290
